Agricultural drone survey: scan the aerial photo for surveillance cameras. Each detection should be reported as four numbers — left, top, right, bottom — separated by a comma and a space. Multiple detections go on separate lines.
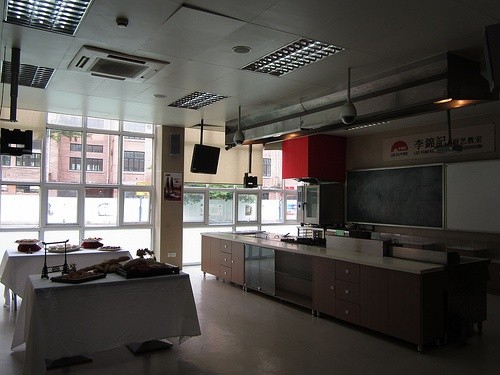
233, 131, 245, 146
340, 103, 358, 124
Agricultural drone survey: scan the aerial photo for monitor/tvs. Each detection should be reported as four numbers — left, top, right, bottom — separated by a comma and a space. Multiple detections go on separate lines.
190, 144, 220, 174
0, 128, 33, 156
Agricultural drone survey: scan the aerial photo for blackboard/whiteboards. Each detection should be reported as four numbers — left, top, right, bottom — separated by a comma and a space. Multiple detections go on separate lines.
343, 163, 449, 231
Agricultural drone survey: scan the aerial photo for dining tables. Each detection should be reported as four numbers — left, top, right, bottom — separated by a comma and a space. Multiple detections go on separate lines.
11, 270, 201, 375
1, 246, 128, 311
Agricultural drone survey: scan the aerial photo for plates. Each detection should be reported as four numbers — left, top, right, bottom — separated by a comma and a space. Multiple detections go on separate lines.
96, 246, 121, 251
45, 244, 80, 253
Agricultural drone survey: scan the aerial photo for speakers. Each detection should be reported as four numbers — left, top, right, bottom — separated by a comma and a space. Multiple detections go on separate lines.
170, 131, 180, 154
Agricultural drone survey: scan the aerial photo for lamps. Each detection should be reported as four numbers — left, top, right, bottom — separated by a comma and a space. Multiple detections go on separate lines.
243, 144, 258, 189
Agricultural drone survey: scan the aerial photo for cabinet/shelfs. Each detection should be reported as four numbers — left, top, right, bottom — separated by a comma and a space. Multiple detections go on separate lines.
451, 255, 490, 343
245, 243, 316, 315
314, 250, 360, 328
201, 232, 220, 280
219, 236, 244, 290
359, 263, 454, 351
